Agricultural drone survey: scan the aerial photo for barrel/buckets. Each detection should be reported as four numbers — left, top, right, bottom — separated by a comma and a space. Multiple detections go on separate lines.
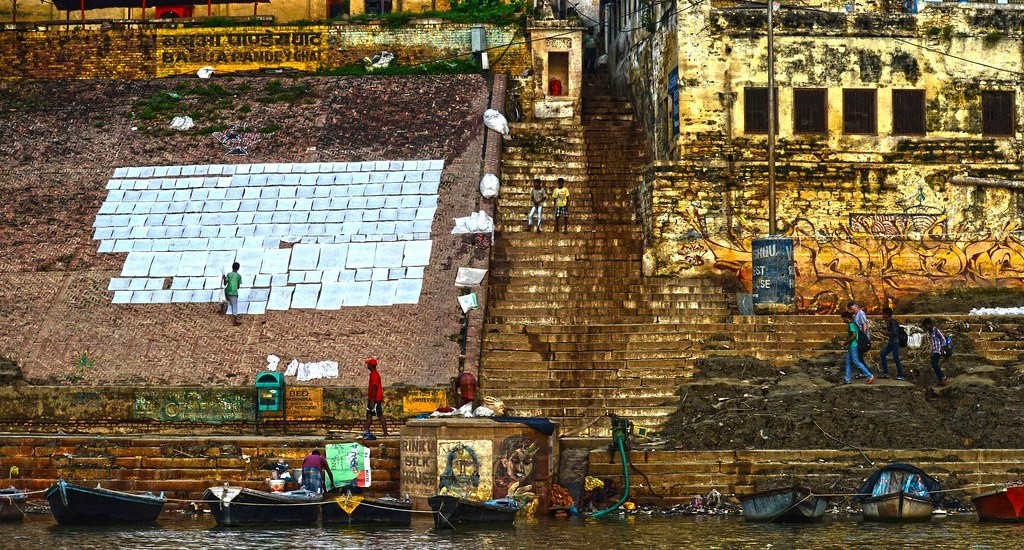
269, 479, 285, 494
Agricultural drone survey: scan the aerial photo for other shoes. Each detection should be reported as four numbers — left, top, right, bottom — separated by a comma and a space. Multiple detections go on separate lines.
892, 376, 906, 381
865, 377, 875, 384
936, 376, 948, 387
553, 229, 559, 232
839, 382, 851, 385
877, 373, 889, 379
233, 322, 244, 326
537, 229, 541, 233
564, 230, 568, 234
524, 228, 532, 232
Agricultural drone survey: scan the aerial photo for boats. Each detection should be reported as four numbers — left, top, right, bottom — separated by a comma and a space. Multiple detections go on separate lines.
45, 478, 168, 526
0, 487, 29, 522
427, 494, 520, 530
970, 482, 1024, 523
855, 465, 944, 523
202, 486, 415, 526
738, 485, 832, 524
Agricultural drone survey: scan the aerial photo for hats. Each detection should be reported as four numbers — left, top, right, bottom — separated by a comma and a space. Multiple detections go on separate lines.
364, 359, 378, 366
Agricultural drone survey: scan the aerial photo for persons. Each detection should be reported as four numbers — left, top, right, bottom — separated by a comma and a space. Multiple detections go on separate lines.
879, 307, 905, 380
841, 311, 874, 383
525, 179, 546, 232
222, 263, 242, 326
922, 318, 947, 384
364, 359, 388, 436
552, 178, 570, 234
301, 450, 336, 493
846, 302, 869, 378
452, 372, 475, 409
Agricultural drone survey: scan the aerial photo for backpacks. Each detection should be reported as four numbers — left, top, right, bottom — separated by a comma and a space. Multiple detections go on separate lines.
849, 322, 871, 352
889, 319, 908, 348
934, 327, 953, 358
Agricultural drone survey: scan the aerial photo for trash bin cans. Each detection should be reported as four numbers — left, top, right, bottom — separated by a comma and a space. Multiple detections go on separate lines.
255, 372, 284, 411
752, 237, 796, 315
